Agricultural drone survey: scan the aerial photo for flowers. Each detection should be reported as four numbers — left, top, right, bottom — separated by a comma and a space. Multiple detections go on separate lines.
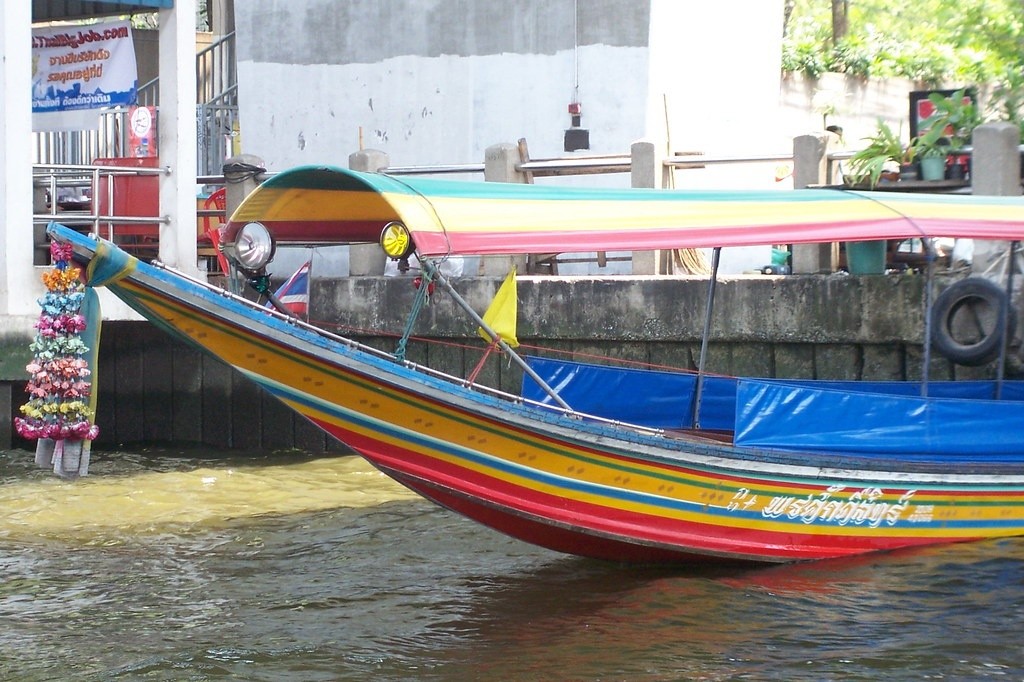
15, 240, 100, 450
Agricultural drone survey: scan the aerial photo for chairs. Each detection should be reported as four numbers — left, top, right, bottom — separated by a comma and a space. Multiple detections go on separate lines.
199, 187, 230, 277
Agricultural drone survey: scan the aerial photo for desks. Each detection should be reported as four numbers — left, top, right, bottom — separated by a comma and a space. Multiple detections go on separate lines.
810, 179, 972, 190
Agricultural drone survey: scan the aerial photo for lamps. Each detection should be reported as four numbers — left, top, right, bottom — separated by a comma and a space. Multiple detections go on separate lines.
380, 221, 417, 275
235, 221, 276, 276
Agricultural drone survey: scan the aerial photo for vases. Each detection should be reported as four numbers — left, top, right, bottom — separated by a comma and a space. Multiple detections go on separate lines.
842, 174, 870, 183
844, 240, 887, 276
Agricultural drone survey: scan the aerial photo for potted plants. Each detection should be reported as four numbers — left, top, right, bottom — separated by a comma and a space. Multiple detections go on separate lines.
920, 144, 947, 181
919, 86, 986, 180
848, 116, 922, 193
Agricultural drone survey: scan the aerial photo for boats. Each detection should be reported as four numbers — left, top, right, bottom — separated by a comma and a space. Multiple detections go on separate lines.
42, 164, 1024, 566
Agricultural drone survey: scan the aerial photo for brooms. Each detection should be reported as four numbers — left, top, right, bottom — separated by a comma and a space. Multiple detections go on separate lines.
662, 90, 712, 274
